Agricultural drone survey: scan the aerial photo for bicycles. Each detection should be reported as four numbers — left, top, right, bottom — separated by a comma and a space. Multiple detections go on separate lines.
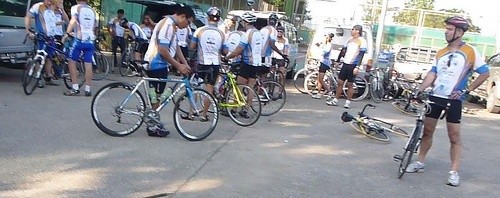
90, 57, 219, 141
292, 58, 430, 117
342, 104, 410, 142
394, 92, 451, 179
20, 28, 147, 95
170, 54, 286, 127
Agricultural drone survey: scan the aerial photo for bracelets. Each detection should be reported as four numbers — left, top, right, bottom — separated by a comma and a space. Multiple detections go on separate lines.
461, 88, 470, 95
65, 32, 69, 36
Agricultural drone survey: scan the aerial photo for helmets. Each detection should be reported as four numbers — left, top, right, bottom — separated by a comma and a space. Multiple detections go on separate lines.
206, 6, 221, 18
269, 14, 278, 23
240, 12, 257, 23
276, 26, 284, 32
119, 17, 128, 27
445, 16, 468, 32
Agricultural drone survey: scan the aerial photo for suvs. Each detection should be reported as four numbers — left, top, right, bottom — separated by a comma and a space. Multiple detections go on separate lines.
126, 0, 223, 33
0, 0, 35, 65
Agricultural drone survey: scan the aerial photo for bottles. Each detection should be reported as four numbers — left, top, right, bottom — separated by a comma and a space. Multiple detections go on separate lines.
158, 86, 173, 103
148, 84, 158, 104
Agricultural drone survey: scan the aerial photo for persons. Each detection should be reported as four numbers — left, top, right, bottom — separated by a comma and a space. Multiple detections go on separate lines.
312, 33, 334, 100
26, 0, 289, 137
326, 24, 367, 108
411, 16, 491, 186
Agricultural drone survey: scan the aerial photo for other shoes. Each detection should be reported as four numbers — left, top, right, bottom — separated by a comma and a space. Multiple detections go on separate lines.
188, 114, 209, 121
447, 171, 460, 186
312, 93, 322, 99
85, 89, 91, 96
326, 98, 338, 106
239, 109, 249, 118
405, 160, 424, 173
127, 70, 139, 76
147, 123, 170, 137
64, 86, 80, 95
182, 112, 198, 120
220, 109, 237, 117
38, 80, 44, 87
344, 100, 350, 108
45, 80, 60, 86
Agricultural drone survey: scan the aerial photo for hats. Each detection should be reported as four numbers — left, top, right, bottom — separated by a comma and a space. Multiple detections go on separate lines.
354, 25, 362, 35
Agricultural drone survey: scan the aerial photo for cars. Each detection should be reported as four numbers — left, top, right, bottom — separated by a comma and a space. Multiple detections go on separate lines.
470, 52, 500, 114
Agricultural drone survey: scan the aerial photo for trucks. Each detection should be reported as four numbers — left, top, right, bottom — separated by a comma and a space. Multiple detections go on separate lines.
393, 46, 438, 77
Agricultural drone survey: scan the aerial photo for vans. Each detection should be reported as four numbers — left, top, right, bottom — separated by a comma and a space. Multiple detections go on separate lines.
219, 8, 301, 79
305, 24, 374, 66
